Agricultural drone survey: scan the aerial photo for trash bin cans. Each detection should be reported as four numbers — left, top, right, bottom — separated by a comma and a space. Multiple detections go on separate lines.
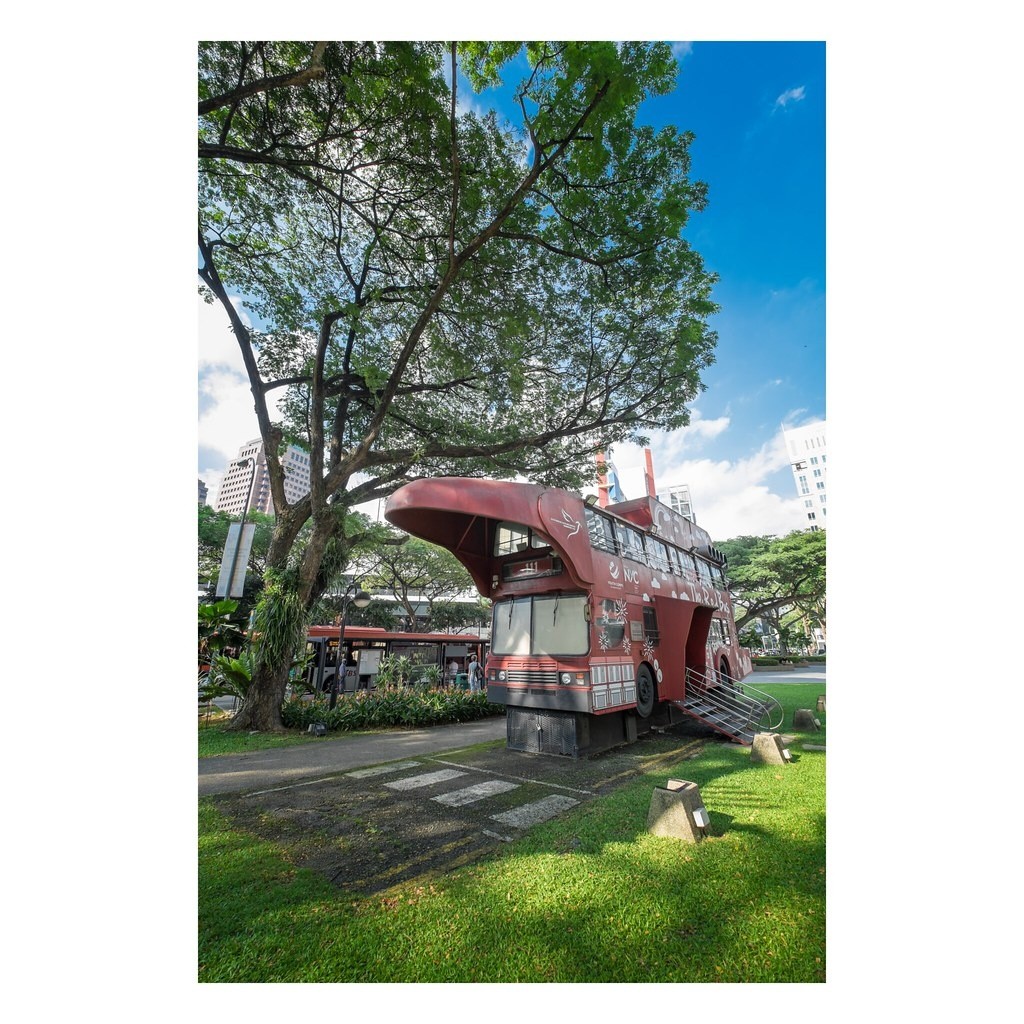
455, 674, 470, 689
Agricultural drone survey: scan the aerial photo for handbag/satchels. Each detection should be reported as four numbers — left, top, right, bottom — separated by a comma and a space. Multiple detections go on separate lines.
475, 663, 483, 679
338, 675, 341, 679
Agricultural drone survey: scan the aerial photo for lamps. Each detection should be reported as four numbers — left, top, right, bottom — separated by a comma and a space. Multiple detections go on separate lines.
719, 560, 728, 568
586, 494, 599, 505
314, 724, 328, 736
689, 546, 698, 554
647, 524, 658, 534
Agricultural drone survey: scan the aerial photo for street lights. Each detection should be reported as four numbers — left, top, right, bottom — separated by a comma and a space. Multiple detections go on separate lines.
330, 583, 371, 712
207, 457, 256, 687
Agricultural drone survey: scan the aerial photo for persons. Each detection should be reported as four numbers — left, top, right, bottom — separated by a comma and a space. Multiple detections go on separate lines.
357, 675, 371, 689
484, 651, 489, 679
446, 658, 458, 680
339, 659, 347, 693
219, 647, 246, 659
468, 655, 483, 690
466, 657, 472, 674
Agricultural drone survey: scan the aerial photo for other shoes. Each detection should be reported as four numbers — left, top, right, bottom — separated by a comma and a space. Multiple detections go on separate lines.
339, 693, 344, 695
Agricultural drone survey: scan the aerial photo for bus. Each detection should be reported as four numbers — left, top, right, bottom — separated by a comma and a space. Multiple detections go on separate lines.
384, 478, 785, 746
199, 630, 264, 696
302, 626, 481, 695
